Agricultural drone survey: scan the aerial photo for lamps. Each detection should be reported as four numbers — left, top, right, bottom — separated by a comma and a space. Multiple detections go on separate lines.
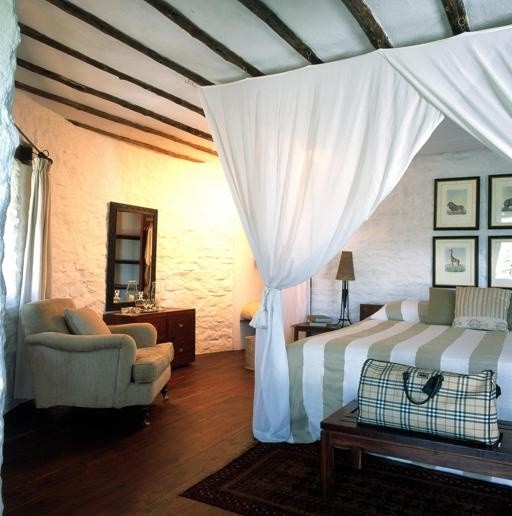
331, 251, 355, 327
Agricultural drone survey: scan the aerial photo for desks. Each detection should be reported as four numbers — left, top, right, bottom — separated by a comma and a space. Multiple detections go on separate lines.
318, 398, 511, 506
290, 321, 343, 342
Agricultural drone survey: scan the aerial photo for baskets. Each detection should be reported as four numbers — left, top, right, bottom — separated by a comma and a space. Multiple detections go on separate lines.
244, 336, 255, 371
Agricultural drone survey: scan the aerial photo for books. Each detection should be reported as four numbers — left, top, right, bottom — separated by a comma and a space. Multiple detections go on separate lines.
306, 320, 328, 327
308, 315, 332, 322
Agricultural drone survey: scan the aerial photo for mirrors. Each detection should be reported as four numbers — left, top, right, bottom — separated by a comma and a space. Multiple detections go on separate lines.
105, 201, 159, 311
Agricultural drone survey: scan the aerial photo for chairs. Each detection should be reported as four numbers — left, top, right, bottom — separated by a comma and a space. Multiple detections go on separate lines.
22, 297, 176, 429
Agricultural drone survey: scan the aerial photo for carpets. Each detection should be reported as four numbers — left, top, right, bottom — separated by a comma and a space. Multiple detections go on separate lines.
177, 438, 512, 513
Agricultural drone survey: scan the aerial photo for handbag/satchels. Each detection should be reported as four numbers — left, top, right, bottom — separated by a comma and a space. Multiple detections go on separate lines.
350, 358, 501, 446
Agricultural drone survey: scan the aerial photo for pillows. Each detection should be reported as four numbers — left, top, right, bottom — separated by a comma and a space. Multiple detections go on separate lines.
429, 287, 512, 331
64, 306, 112, 336
451, 284, 511, 333
371, 299, 430, 322
240, 299, 260, 320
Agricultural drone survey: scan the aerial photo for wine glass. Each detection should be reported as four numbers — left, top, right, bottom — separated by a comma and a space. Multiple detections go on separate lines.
141, 298, 155, 311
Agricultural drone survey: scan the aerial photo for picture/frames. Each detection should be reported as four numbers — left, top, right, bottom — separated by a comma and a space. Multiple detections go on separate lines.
430, 175, 480, 231
486, 172, 512, 229
430, 234, 480, 289
487, 235, 512, 291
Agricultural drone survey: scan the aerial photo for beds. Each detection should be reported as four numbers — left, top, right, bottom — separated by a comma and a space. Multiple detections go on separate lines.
286, 302, 512, 443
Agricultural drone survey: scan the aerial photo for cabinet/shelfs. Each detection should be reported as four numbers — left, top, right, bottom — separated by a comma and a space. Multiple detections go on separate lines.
102, 304, 196, 369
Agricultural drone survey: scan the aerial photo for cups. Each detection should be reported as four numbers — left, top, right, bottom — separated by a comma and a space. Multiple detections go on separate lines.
121, 306, 136, 313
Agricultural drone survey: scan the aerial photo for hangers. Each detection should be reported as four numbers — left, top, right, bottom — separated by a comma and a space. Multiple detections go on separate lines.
143, 217, 153, 231
